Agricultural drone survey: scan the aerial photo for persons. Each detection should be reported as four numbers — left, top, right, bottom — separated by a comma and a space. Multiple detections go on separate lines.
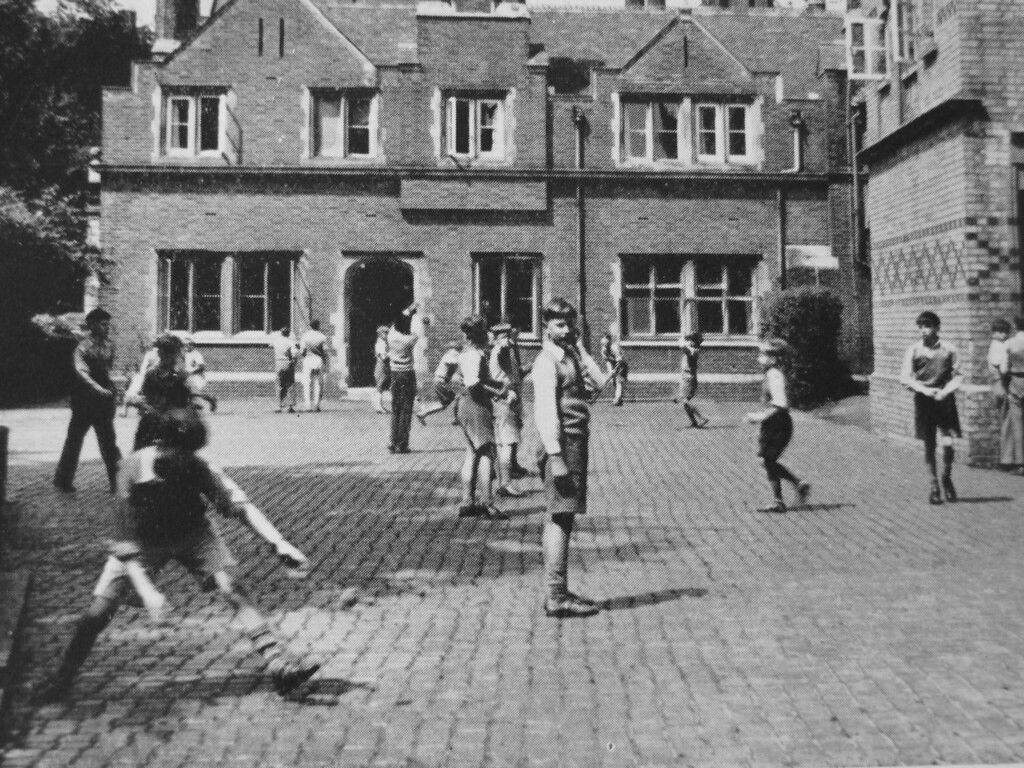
53, 309, 122, 494
457, 313, 532, 519
270, 319, 327, 413
375, 326, 391, 414
988, 311, 1024, 475
600, 334, 628, 407
745, 336, 811, 513
118, 331, 214, 449
42, 411, 321, 693
532, 298, 607, 617
680, 334, 708, 428
416, 341, 462, 426
902, 311, 964, 504
388, 304, 418, 453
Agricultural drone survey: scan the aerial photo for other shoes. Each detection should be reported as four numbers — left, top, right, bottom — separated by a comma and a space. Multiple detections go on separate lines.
499, 487, 531, 497
929, 488, 941, 505
757, 502, 787, 513
687, 419, 709, 428
505, 468, 529, 479
459, 506, 487, 517
210, 395, 219, 413
271, 661, 320, 697
388, 445, 411, 451
29, 677, 70, 705
275, 408, 283, 413
417, 412, 424, 421
482, 511, 511, 521
941, 477, 957, 503
543, 592, 599, 616
288, 408, 298, 413
52, 477, 79, 494
797, 483, 810, 504
